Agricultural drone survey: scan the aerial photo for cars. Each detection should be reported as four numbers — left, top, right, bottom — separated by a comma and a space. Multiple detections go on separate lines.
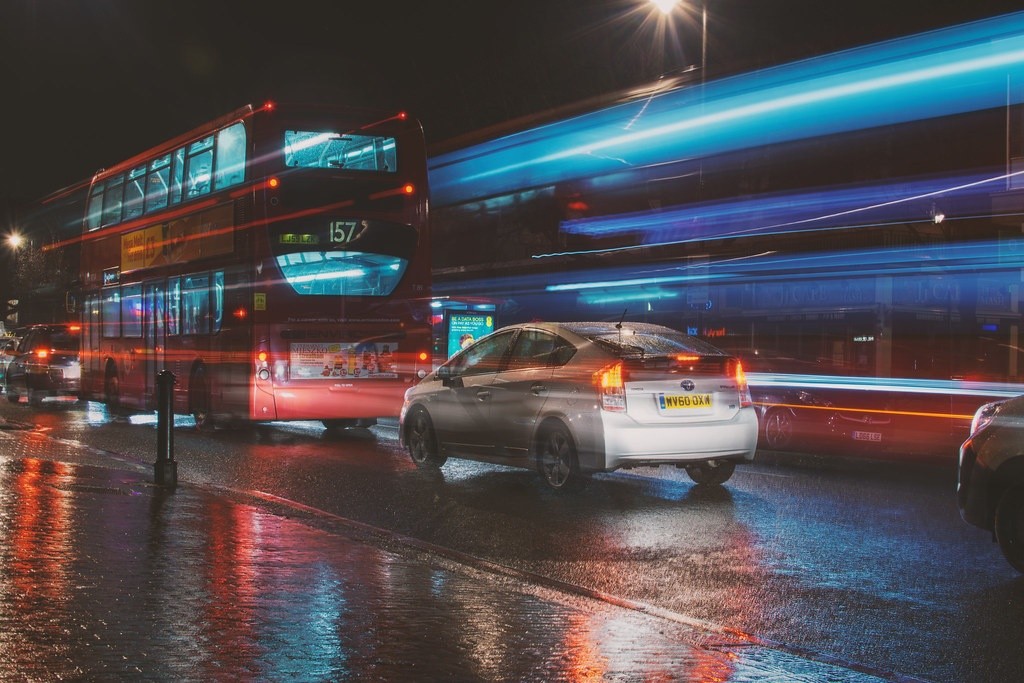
954, 393, 1023, 578
5, 319, 81, 408
396, 323, 758, 495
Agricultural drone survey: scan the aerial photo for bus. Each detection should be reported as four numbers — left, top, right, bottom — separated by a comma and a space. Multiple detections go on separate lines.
75, 99, 441, 440
430, 13, 1024, 466
